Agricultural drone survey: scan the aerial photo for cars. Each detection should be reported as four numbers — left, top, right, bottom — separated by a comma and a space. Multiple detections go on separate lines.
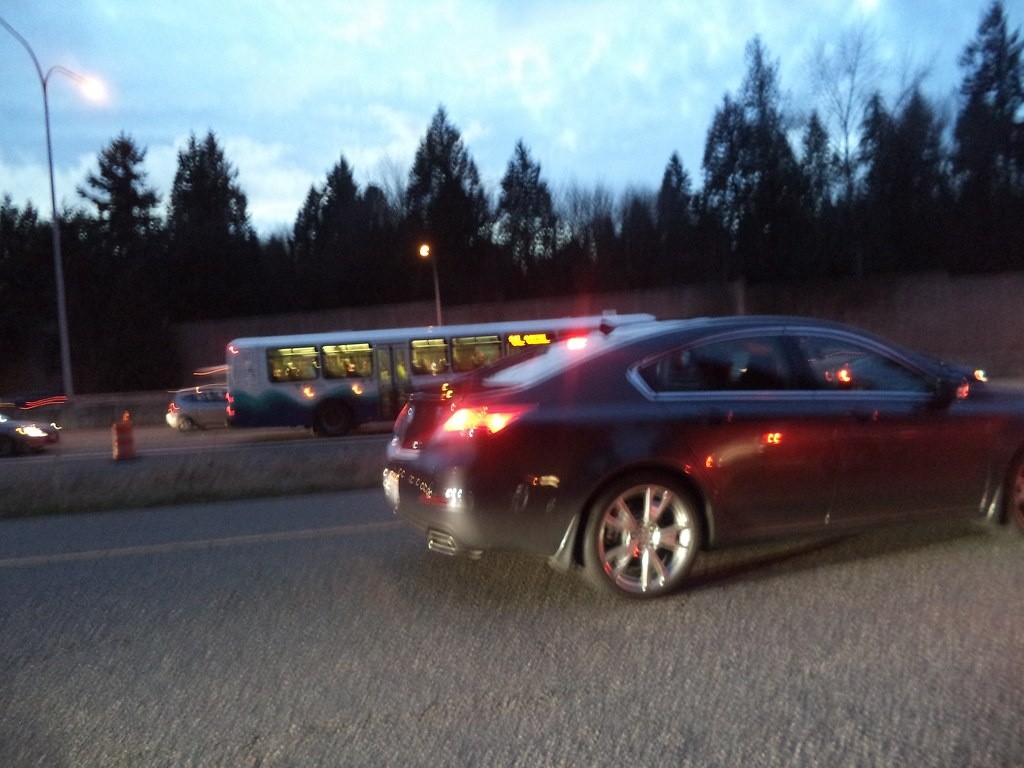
0, 415, 61, 453
382, 316, 1024, 601
166, 383, 229, 434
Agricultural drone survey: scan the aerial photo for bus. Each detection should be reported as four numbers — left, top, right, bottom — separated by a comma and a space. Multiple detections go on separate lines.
223, 314, 657, 438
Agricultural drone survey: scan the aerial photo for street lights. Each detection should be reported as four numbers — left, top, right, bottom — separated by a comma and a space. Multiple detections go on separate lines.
41, 64, 110, 406
419, 244, 444, 329
